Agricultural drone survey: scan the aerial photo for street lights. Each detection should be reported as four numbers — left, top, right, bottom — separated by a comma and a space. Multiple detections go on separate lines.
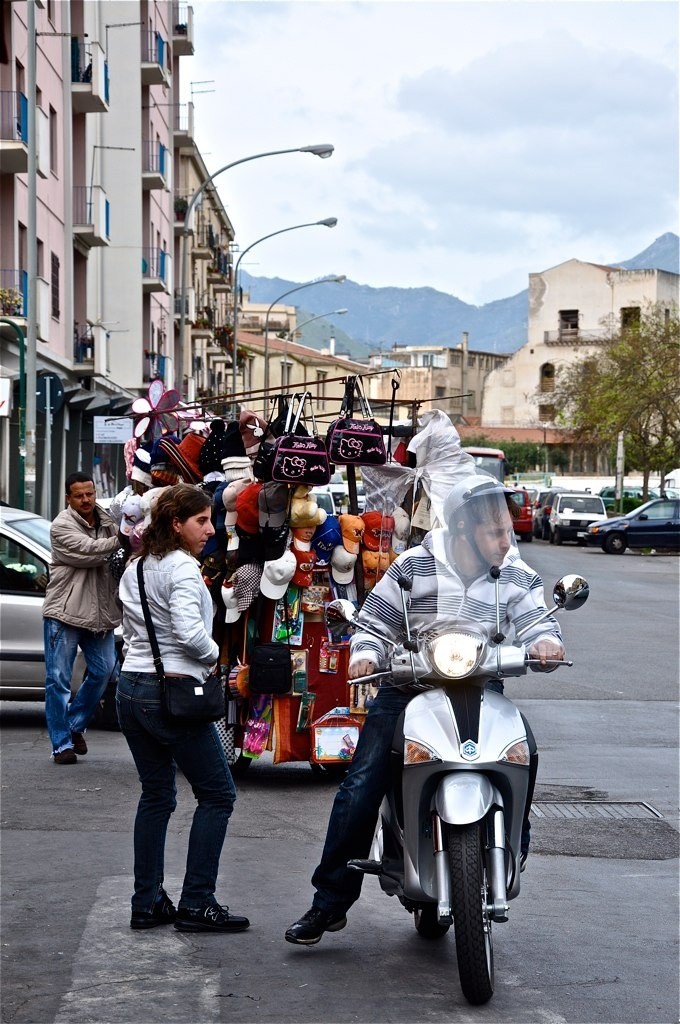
282, 308, 350, 396
231, 216, 340, 422
176, 141, 338, 400
264, 273, 348, 421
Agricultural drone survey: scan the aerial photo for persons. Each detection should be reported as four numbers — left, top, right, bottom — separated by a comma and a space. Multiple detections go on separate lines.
41, 470, 123, 765
111, 486, 253, 934
284, 473, 564, 944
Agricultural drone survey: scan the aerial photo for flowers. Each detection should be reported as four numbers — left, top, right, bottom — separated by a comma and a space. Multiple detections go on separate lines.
0, 287, 24, 316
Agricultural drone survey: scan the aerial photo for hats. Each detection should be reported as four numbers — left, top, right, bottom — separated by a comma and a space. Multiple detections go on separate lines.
118, 412, 419, 623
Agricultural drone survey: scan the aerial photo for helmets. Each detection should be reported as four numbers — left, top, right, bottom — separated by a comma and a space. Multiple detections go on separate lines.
443, 473, 518, 526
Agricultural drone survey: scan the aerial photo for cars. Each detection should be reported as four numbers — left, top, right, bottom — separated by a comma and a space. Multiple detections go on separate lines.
582, 497, 680, 555
307, 473, 367, 516
0, 503, 123, 732
504, 467, 680, 545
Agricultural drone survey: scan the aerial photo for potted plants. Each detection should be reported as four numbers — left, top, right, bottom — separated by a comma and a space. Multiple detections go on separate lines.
174, 197, 188, 221
197, 386, 230, 420
176, 24, 186, 35
195, 319, 250, 370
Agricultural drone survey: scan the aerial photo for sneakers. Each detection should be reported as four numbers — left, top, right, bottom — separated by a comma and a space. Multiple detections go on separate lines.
285, 901, 349, 944
54, 750, 76, 764
174, 896, 250, 933
70, 728, 88, 755
519, 852, 527, 872
131, 895, 178, 928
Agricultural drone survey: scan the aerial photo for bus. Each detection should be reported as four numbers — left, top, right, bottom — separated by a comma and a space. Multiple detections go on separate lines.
462, 445, 507, 483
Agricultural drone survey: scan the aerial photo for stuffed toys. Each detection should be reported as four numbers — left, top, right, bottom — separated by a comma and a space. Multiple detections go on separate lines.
286, 482, 327, 529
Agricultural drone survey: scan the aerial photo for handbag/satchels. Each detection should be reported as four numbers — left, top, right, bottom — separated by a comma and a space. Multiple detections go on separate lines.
158, 664, 224, 722
252, 373, 388, 486
250, 643, 293, 694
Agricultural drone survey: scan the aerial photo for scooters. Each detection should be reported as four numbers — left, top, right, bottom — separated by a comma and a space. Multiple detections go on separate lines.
324, 572, 592, 1005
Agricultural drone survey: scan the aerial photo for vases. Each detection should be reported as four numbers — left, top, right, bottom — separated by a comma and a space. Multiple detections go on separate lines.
2, 307, 15, 316
